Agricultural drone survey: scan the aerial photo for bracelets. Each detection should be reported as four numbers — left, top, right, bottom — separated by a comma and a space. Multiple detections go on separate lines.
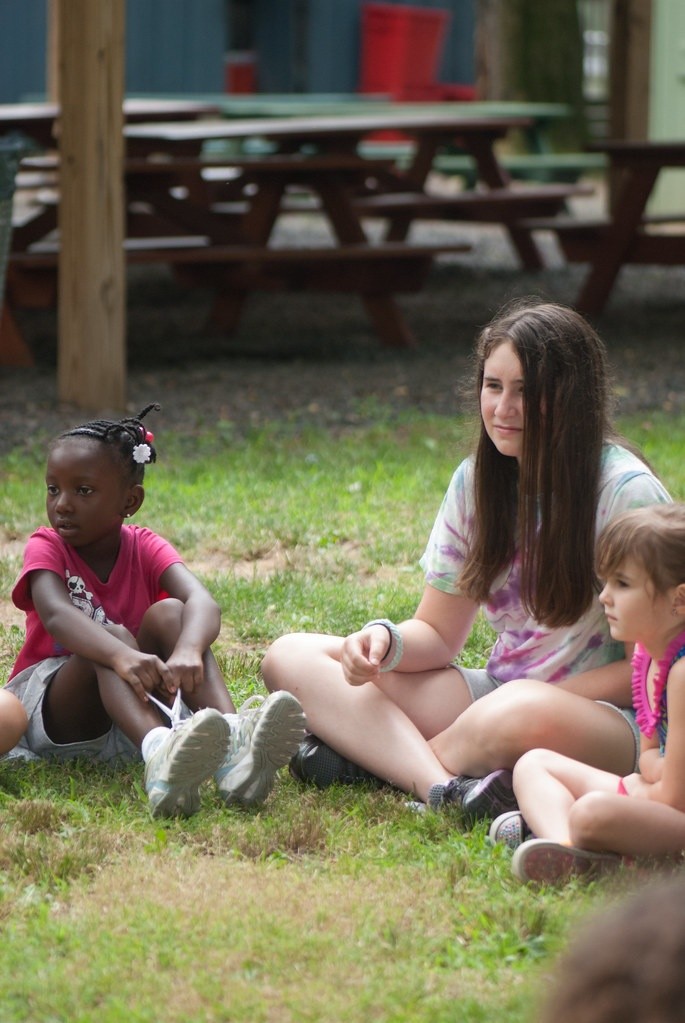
362, 619, 403, 672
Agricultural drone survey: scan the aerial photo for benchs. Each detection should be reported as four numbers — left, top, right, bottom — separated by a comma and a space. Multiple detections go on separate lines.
18, 155, 396, 181
325, 187, 591, 207
1, 237, 472, 267
521, 214, 685, 241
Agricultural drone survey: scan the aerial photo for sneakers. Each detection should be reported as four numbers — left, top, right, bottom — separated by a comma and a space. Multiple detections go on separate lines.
144, 687, 231, 821
489, 810, 539, 853
287, 727, 387, 791
509, 838, 629, 888
214, 689, 307, 811
427, 768, 521, 834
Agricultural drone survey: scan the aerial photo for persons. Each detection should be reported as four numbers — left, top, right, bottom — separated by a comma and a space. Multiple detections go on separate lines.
533, 882, 685, 1023
0, 688, 28, 756
489, 505, 685, 891
3, 402, 306, 821
261, 305, 675, 837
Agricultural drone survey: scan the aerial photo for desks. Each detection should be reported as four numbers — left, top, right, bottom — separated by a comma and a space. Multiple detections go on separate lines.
575, 137, 685, 325
116, 103, 574, 326
0, 93, 392, 135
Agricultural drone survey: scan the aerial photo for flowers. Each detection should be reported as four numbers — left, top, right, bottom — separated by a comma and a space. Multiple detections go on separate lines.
132, 443, 152, 464
